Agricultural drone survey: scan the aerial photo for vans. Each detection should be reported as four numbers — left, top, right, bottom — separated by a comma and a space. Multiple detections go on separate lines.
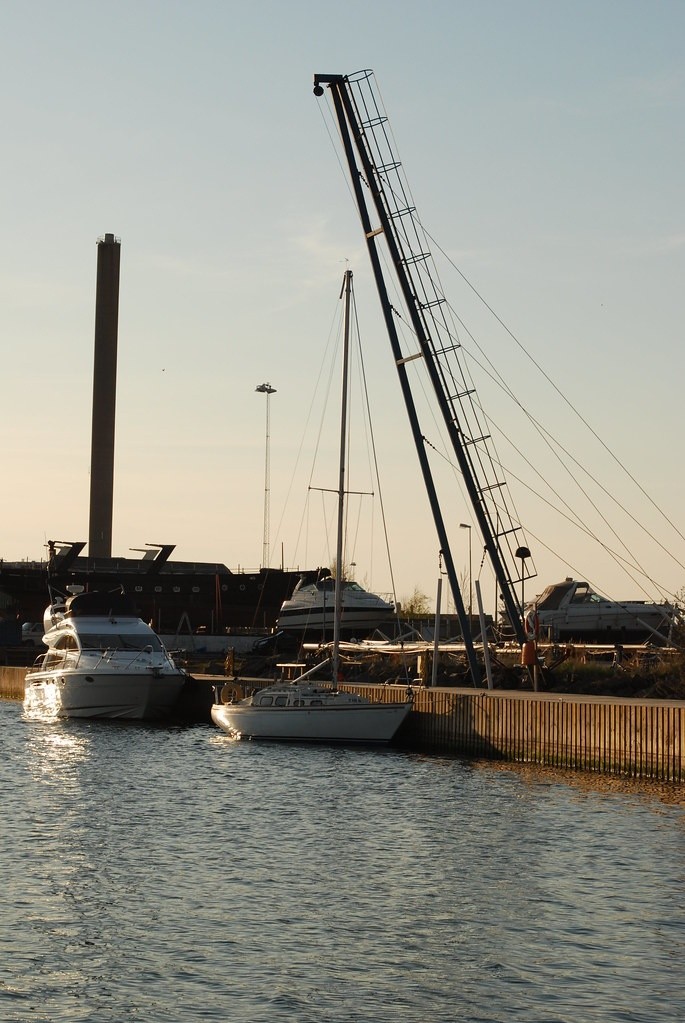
22, 622, 44, 648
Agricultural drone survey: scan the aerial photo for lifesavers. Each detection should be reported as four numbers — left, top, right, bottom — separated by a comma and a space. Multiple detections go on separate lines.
524, 611, 539, 640
221, 683, 243, 704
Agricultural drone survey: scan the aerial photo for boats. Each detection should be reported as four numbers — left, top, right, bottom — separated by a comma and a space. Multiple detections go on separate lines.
498, 577, 674, 643
277, 567, 394, 633
23, 584, 198, 726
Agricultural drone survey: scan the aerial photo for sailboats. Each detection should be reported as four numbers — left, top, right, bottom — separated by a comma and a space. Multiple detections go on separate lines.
207, 271, 425, 751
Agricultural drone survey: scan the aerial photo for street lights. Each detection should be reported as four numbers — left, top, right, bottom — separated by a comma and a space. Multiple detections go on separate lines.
514, 547, 531, 619
254, 382, 277, 569
458, 522, 473, 637
